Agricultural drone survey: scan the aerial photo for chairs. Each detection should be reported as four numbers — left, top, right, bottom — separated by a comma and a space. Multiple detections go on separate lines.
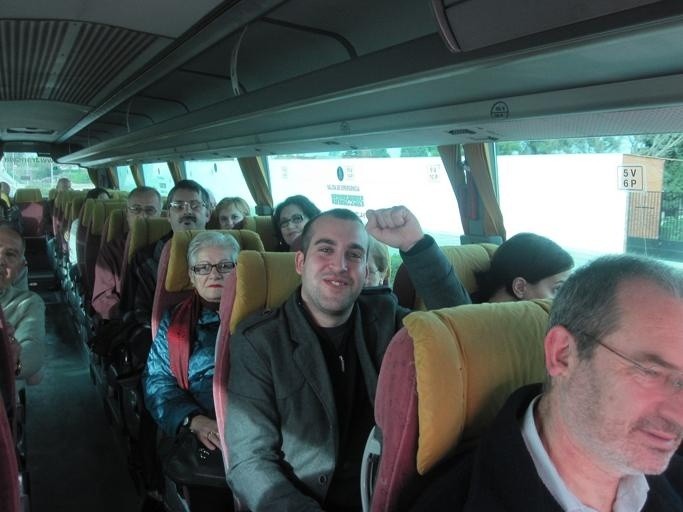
0, 189, 553, 511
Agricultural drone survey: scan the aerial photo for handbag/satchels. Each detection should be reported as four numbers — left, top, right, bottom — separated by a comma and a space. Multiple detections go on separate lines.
92, 309, 145, 346
161, 382, 227, 487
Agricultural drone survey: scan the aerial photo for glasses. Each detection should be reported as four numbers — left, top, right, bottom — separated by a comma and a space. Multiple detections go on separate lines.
578, 329, 682, 396
170, 200, 207, 209
127, 205, 157, 216
278, 213, 307, 227
190, 261, 237, 276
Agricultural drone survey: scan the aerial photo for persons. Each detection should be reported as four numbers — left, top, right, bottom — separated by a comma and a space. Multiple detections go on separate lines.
91, 180, 322, 511
68, 188, 111, 269
225, 205, 471, 511
470, 233, 575, 305
1, 182, 24, 234
390, 251, 683, 512
41, 179, 71, 240
1, 222, 45, 405
